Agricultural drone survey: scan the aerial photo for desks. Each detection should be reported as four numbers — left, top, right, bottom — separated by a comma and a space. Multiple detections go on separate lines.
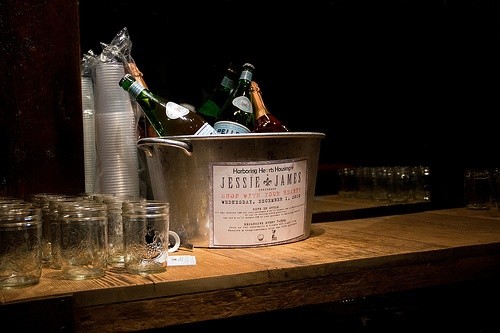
0, 187, 500, 333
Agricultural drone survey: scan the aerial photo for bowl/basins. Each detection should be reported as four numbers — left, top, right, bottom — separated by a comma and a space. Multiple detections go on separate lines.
136, 132, 326, 248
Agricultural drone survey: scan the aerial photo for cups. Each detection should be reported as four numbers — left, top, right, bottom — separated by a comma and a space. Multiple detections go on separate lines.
463, 170, 500, 210
338, 166, 430, 201
0, 193, 146, 287
122, 201, 180, 273
82, 64, 138, 197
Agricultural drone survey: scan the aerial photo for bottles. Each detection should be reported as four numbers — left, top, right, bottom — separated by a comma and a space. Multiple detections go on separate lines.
215, 63, 255, 135
195, 69, 236, 129
248, 81, 289, 132
119, 73, 219, 136
